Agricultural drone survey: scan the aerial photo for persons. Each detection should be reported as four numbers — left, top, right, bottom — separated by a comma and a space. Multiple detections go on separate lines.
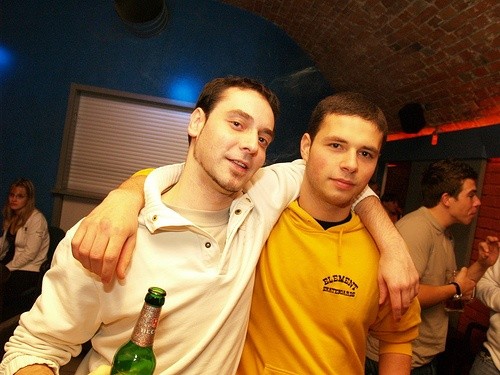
71, 92, 422, 375
0, 178, 50, 323
365, 159, 500, 375
470, 252, 500, 375
368, 180, 404, 223
0, 75, 419, 375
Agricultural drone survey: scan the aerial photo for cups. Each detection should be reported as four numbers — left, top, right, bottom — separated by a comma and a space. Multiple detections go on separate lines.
444, 270, 475, 313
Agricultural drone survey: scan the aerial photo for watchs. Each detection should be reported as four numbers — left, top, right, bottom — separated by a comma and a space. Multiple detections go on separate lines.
448, 281, 463, 301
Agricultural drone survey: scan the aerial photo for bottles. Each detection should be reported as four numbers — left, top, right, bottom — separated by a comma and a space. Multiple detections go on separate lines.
110, 288, 167, 375
